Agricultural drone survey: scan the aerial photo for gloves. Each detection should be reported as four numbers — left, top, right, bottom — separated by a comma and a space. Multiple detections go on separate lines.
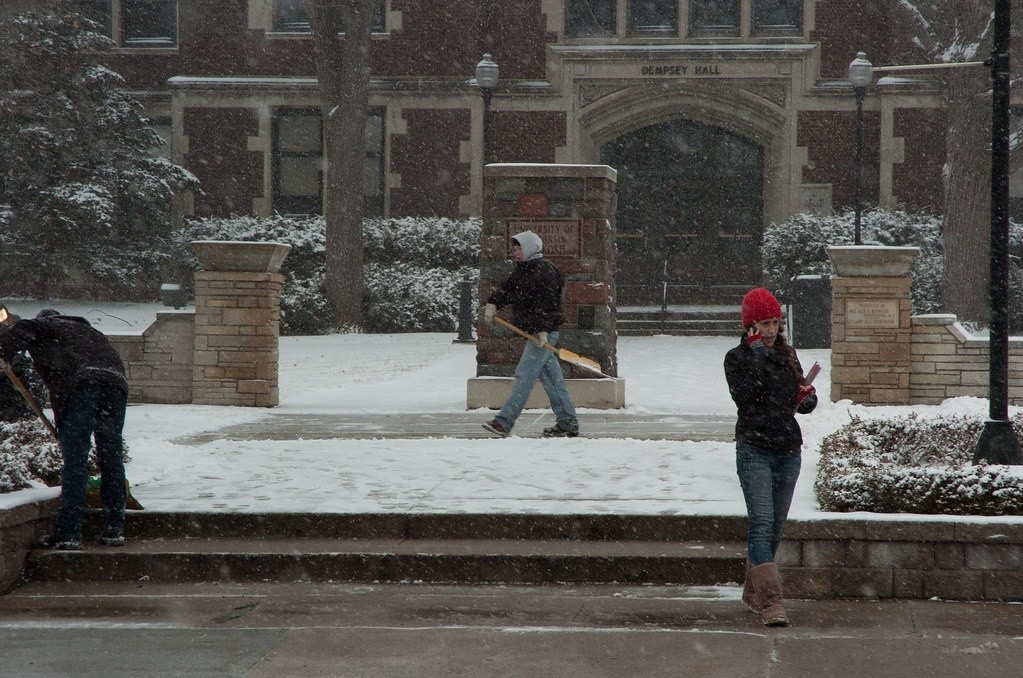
483, 303, 496, 322
534, 332, 548, 349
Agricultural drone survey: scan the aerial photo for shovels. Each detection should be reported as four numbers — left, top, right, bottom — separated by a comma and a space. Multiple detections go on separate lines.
0, 358, 145, 511
493, 316, 618, 381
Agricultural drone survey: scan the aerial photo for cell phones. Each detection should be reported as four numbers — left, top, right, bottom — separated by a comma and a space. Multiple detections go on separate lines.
745, 327, 759, 338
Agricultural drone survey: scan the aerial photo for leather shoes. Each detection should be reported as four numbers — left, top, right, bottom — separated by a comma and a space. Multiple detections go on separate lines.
33, 533, 82, 551
94, 526, 125, 545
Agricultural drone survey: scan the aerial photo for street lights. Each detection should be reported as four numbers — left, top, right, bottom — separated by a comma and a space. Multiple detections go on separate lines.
847, 50, 872, 245
473, 52, 501, 165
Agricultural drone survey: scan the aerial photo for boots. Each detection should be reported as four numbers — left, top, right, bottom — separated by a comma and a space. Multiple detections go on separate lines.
750, 562, 790, 625
741, 562, 763, 615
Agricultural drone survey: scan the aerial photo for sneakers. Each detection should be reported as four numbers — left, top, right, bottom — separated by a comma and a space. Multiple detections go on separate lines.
480, 420, 508, 436
544, 423, 579, 437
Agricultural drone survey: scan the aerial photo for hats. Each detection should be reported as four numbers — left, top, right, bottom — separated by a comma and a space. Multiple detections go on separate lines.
741, 287, 783, 327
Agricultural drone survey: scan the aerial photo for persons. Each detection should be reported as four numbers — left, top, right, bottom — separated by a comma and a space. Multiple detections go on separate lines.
482, 230, 582, 439
722, 286, 818, 629
0, 307, 131, 552
